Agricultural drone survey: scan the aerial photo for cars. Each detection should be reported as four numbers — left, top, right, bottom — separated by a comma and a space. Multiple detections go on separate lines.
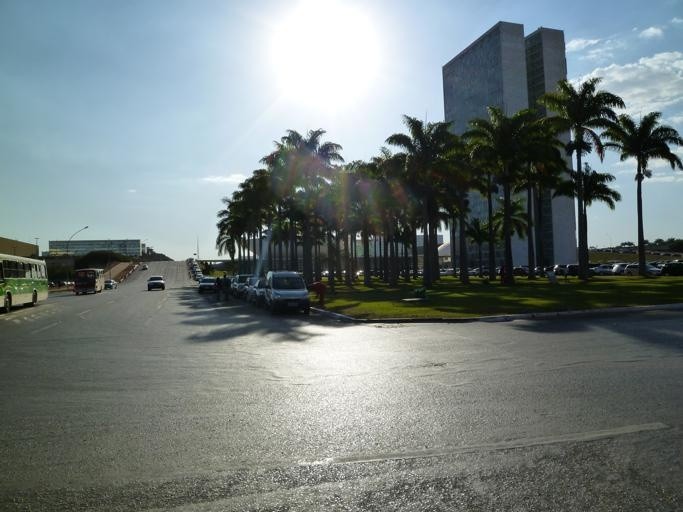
146, 276, 164, 291
191, 261, 310, 315
104, 280, 116, 290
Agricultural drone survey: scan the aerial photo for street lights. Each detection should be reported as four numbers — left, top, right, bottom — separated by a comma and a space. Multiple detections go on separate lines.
66, 225, 88, 288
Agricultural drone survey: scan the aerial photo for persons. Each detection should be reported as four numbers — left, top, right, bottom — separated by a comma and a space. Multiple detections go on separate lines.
306, 282, 325, 306
219, 274, 230, 302
213, 276, 220, 300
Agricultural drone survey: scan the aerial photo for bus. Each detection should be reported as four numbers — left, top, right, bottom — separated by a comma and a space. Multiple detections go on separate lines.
73, 267, 104, 296
0, 254, 48, 313
